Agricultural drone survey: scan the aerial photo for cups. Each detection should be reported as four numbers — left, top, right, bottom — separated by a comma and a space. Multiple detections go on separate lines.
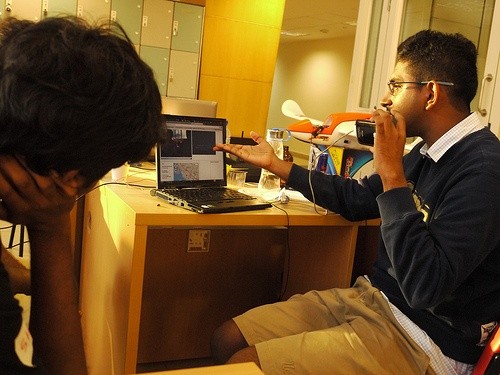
227, 170, 246, 190
257, 129, 291, 202
111, 163, 129, 182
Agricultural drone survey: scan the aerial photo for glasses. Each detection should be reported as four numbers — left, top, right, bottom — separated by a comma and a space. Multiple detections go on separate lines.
387, 79, 455, 93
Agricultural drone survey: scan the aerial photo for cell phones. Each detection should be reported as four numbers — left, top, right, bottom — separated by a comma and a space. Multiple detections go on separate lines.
356, 119, 376, 146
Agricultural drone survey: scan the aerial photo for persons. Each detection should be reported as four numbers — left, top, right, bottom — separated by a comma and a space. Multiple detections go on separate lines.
0, 16, 163, 375
201, 28, 500, 375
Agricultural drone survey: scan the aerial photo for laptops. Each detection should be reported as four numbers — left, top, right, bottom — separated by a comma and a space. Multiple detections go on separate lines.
152, 114, 272, 215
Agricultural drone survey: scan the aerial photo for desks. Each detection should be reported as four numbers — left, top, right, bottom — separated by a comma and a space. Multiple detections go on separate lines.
75, 165, 382, 375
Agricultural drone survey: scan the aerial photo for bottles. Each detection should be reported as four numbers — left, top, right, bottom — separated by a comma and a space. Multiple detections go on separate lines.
280, 146, 294, 189
